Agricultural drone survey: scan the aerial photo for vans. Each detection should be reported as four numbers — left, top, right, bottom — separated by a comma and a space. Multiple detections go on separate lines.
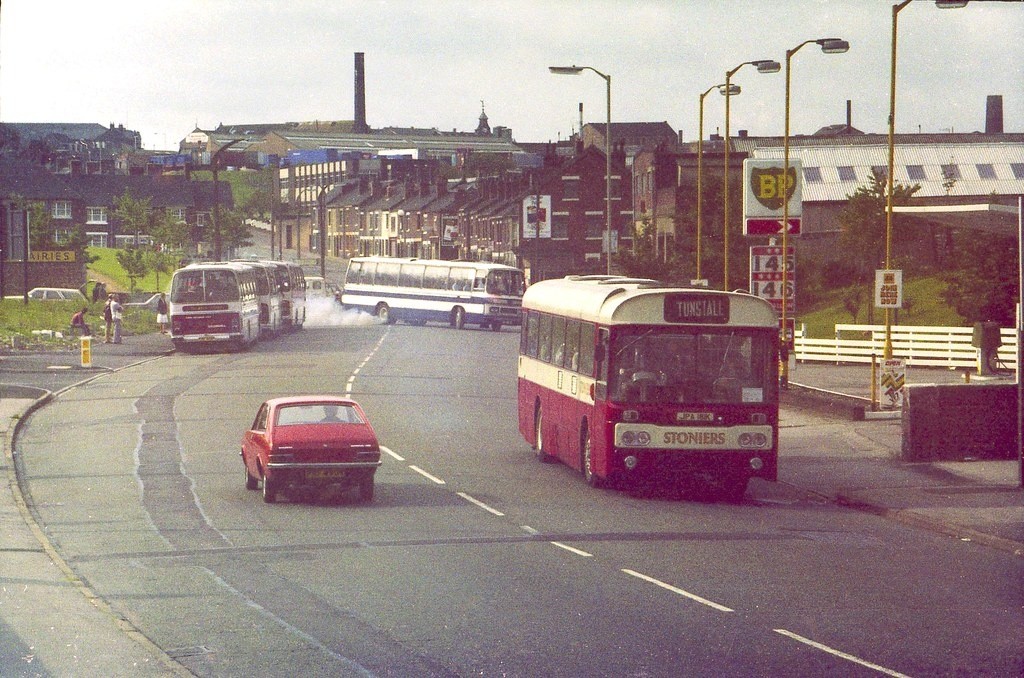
304, 277, 325, 298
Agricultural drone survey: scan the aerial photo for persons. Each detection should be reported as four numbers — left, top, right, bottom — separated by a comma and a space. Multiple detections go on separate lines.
92, 282, 124, 344
71, 308, 91, 336
156, 292, 168, 335
319, 405, 347, 423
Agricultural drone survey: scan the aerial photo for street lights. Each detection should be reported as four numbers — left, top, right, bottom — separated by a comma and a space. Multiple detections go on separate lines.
548, 65, 614, 277
724, 59, 782, 292
883, 0, 969, 367
781, 37, 849, 389
695, 83, 741, 286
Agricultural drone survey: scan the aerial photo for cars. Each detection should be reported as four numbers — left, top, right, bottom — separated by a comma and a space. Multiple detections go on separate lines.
244, 396, 382, 503
3, 280, 98, 302
122, 292, 170, 312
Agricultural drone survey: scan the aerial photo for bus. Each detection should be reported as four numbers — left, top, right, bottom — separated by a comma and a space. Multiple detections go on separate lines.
233, 260, 283, 341
341, 258, 525, 331
170, 262, 260, 352
264, 260, 309, 332
517, 275, 789, 499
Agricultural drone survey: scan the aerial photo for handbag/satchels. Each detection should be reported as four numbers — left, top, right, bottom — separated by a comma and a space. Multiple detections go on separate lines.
116, 312, 122, 320
